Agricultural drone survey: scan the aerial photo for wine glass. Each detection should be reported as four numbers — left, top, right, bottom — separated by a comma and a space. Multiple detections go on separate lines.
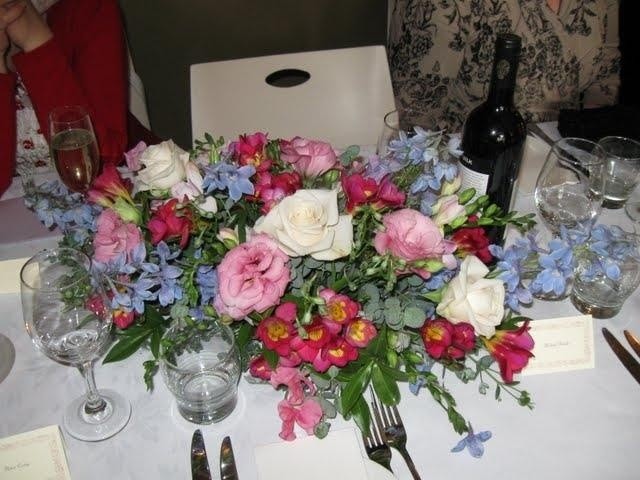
19, 247, 132, 441
45, 106, 105, 216
531, 136, 606, 302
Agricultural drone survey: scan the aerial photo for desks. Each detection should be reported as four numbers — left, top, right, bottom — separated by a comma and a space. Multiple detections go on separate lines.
0, 168, 640, 478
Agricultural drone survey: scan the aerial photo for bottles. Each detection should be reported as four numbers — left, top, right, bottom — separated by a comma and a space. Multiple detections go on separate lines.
459, 34, 527, 271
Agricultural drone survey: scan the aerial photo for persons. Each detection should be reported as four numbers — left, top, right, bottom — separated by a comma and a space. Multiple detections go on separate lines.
0, 1, 163, 203
388, 0, 622, 142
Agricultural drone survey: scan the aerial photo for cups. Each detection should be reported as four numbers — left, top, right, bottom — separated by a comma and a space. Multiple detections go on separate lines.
157, 317, 241, 424
380, 109, 436, 157
594, 134, 639, 211
573, 227, 640, 317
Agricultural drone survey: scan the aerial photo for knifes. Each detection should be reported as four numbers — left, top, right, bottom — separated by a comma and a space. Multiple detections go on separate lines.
601, 327, 639, 383
190, 428, 239, 479
526, 120, 593, 180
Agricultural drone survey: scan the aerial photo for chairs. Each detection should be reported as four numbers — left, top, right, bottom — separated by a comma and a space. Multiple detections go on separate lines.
189, 44, 399, 160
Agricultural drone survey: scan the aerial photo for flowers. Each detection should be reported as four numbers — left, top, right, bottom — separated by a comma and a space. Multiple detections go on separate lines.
17, 126, 640, 459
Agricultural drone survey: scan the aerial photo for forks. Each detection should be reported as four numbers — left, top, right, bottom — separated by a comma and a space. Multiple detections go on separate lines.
354, 384, 421, 480
